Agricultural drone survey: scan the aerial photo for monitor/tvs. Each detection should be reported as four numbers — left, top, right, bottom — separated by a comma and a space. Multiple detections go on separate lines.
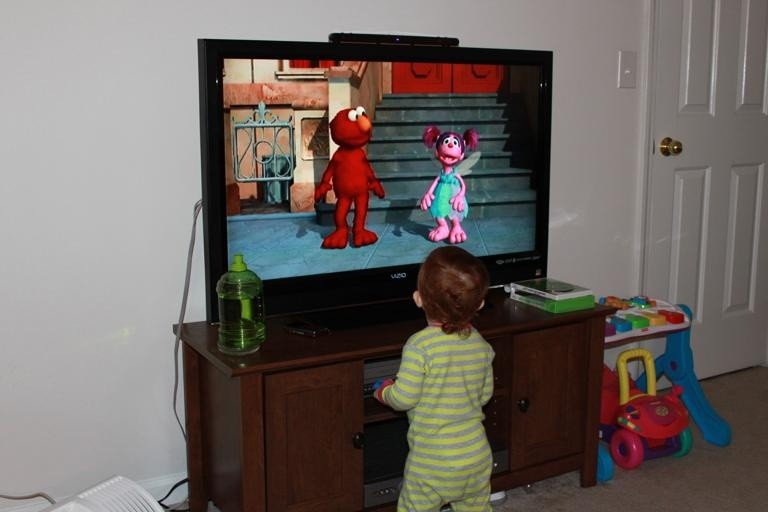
197, 37, 554, 339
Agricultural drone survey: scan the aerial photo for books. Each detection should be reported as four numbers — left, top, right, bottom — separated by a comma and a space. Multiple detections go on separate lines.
510, 289, 595, 315
508, 276, 593, 301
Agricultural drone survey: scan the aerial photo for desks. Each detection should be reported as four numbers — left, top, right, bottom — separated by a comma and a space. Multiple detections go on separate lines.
172, 277, 621, 510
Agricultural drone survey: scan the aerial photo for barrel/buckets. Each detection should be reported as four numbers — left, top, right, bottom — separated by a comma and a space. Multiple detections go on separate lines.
215, 255, 266, 354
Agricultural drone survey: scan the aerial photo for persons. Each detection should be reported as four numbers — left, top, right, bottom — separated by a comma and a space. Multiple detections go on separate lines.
372, 246, 497, 512
